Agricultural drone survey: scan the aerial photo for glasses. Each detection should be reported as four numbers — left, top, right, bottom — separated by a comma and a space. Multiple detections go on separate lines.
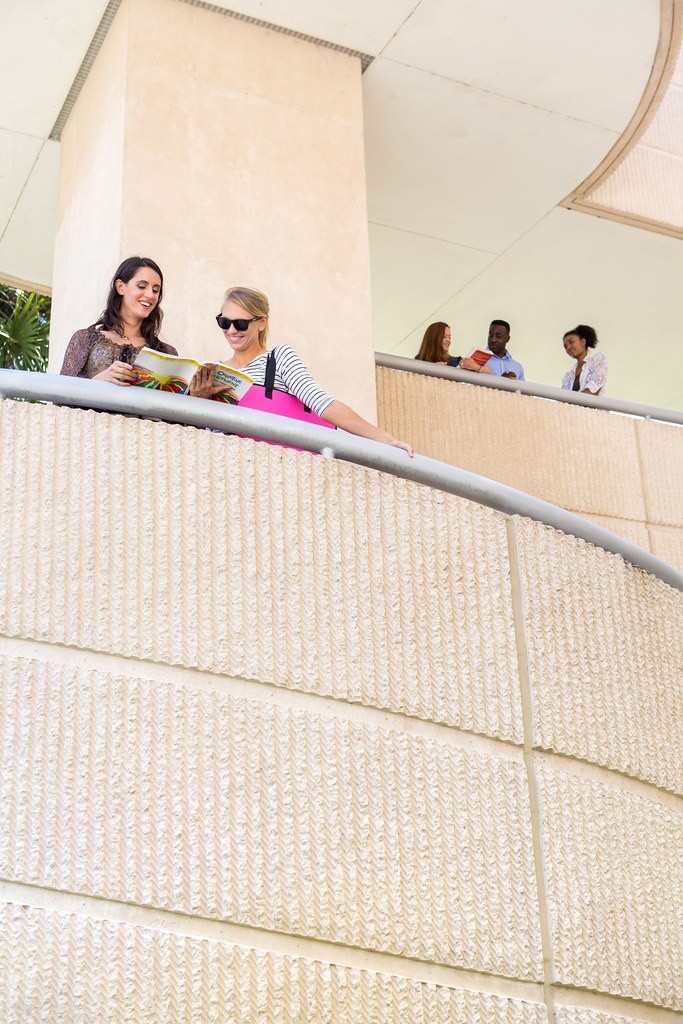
215, 313, 261, 331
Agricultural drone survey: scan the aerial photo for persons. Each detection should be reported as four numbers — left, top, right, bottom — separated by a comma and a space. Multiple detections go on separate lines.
484, 319, 525, 381
54, 257, 234, 428
559, 325, 610, 412
213, 286, 415, 460
413, 322, 491, 379
